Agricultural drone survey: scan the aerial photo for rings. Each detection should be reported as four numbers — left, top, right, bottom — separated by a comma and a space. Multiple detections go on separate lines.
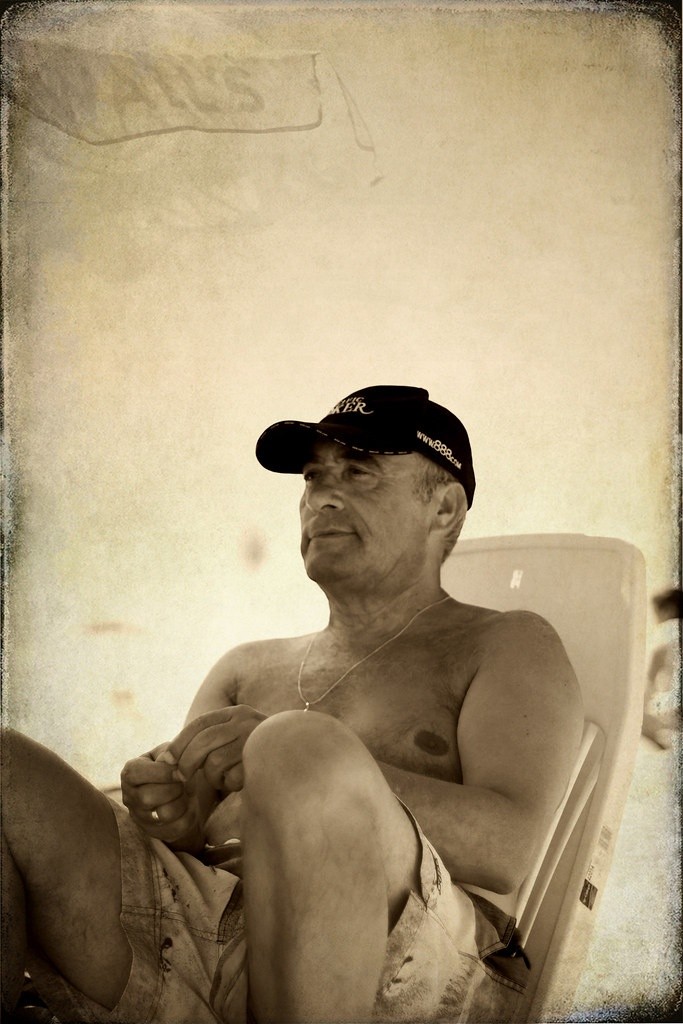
151, 810, 160, 822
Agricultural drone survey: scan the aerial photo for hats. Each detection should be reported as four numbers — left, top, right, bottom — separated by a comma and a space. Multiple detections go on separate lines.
256, 385, 477, 511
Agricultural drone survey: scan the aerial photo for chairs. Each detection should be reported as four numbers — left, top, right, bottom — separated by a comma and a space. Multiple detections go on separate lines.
439, 533, 648, 1023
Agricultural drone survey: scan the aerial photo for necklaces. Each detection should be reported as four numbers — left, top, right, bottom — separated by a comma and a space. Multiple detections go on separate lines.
296, 593, 452, 713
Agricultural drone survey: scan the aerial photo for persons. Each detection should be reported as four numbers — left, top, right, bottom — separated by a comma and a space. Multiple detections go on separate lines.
0, 384, 585, 1024
641, 588, 683, 753
